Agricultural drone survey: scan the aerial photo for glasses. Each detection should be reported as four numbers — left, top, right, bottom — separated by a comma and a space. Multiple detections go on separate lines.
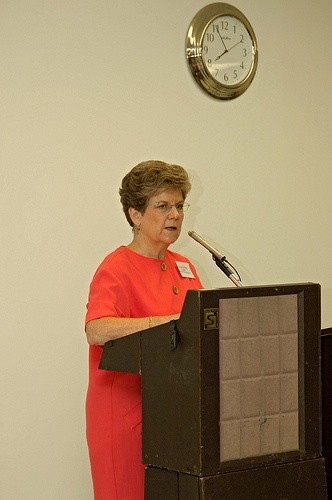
151, 200, 191, 212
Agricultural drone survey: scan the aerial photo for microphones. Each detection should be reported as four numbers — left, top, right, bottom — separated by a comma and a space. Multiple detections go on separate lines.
187, 231, 247, 287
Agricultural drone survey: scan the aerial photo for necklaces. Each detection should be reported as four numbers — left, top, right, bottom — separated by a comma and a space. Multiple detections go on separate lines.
129, 242, 165, 259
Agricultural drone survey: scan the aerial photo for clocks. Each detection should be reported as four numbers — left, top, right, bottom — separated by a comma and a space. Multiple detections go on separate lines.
185, 3, 260, 101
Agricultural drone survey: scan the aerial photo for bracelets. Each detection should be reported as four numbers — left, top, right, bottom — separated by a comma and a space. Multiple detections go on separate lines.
148, 315, 154, 328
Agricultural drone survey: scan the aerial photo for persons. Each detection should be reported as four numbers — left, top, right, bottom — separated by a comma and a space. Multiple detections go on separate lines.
83, 159, 209, 500
